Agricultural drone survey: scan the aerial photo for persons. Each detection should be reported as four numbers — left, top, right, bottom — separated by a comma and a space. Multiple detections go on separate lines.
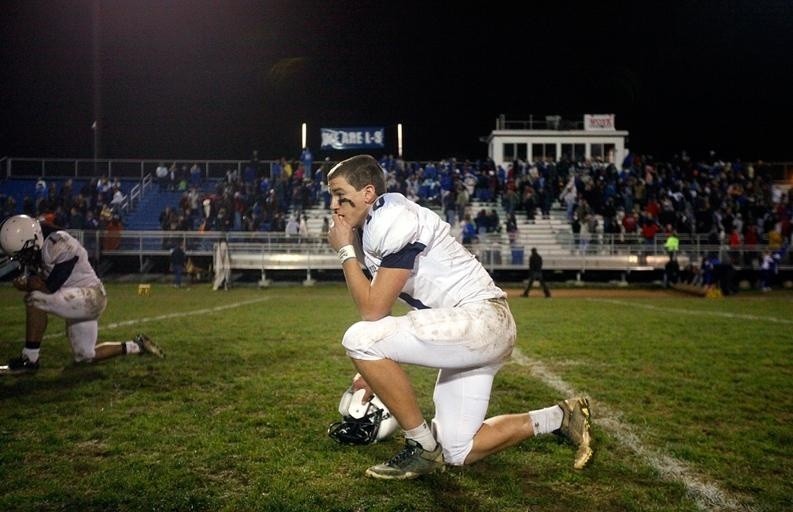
377, 153, 792, 283
0, 213, 168, 383
319, 153, 597, 483
518, 246, 551, 297
1, 152, 330, 288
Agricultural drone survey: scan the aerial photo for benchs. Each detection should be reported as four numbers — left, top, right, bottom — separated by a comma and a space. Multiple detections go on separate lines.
0, 143, 590, 257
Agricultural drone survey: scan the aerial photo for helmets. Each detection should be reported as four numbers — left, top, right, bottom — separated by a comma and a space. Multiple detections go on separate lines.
335, 372, 399, 446
0, 213, 43, 258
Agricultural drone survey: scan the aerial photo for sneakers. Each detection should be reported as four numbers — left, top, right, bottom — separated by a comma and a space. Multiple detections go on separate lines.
362, 435, 447, 483
554, 393, 598, 471
131, 333, 165, 362
0, 355, 41, 376
